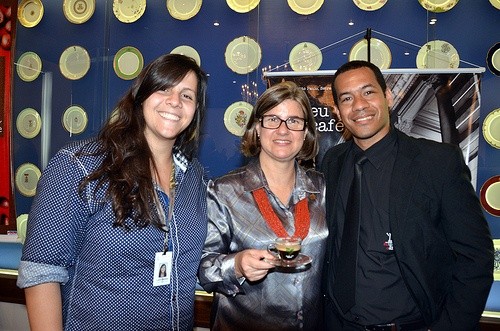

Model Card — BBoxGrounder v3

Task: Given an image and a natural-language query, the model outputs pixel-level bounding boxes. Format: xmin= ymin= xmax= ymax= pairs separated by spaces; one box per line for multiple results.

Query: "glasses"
xmin=258 ymin=115 xmax=310 ymax=131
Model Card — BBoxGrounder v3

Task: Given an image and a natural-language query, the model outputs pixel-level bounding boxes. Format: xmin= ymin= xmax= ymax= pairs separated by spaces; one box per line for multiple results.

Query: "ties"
xmin=333 ymin=147 xmax=369 ymax=313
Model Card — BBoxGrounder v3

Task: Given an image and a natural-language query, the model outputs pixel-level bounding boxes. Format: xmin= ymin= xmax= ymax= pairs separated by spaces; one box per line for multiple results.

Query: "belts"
xmin=323 ymin=290 xmax=425 ymax=331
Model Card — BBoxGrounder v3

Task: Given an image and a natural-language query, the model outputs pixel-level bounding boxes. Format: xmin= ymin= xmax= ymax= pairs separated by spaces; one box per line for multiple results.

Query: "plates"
xmin=486 ymin=42 xmax=500 ymax=77
xmin=264 ymin=253 xmax=311 ymax=268
xmin=16 ymin=52 xmax=42 ymax=82
xmin=167 ymin=0 xmax=202 ymax=21
xmin=59 ymin=46 xmax=90 ymax=80
xmin=17 ymin=214 xmax=31 ymax=245
xmin=170 ymin=45 xmax=200 ymax=68
xmin=354 ymin=1 xmax=388 ymax=11
xmin=491 ymin=238 xmax=500 ymax=281
xmin=227 ymin=0 xmax=261 ymax=13
xmin=288 ymin=42 xmax=323 ymax=72
xmin=16 ymin=108 xmax=42 ymax=139
xmin=60 ymin=104 xmax=88 ymax=136
xmin=416 ymin=41 xmax=459 ymax=69
xmin=224 ymin=101 xmax=254 ymax=136
xmin=482 ymin=108 xmax=500 ymax=149
xmin=14 ymin=163 xmax=41 ymax=197
xmin=17 ymin=0 xmax=44 ymax=28
xmin=480 ymin=176 xmax=500 ymax=218
xmin=419 ymin=0 xmax=459 ymax=13
xmin=113 ymin=0 xmax=146 ymax=23
xmin=63 ymin=0 xmax=95 ymax=25
xmin=112 ymin=46 xmax=144 ymax=80
xmin=224 ymin=36 xmax=262 ymax=74
xmin=348 ymin=37 xmax=391 ymax=70
xmin=287 ymin=0 xmax=324 ymax=15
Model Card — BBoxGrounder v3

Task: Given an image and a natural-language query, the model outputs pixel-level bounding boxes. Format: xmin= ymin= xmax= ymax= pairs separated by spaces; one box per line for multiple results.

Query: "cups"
xmin=267 ymin=236 xmax=301 ymax=263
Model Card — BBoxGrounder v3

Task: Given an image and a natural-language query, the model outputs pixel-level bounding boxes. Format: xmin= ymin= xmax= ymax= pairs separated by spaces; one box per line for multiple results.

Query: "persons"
xmin=199 ymin=81 xmax=329 ymax=331
xmin=17 ymin=53 xmax=206 ymax=330
xmin=318 ymin=60 xmax=495 ymax=331
